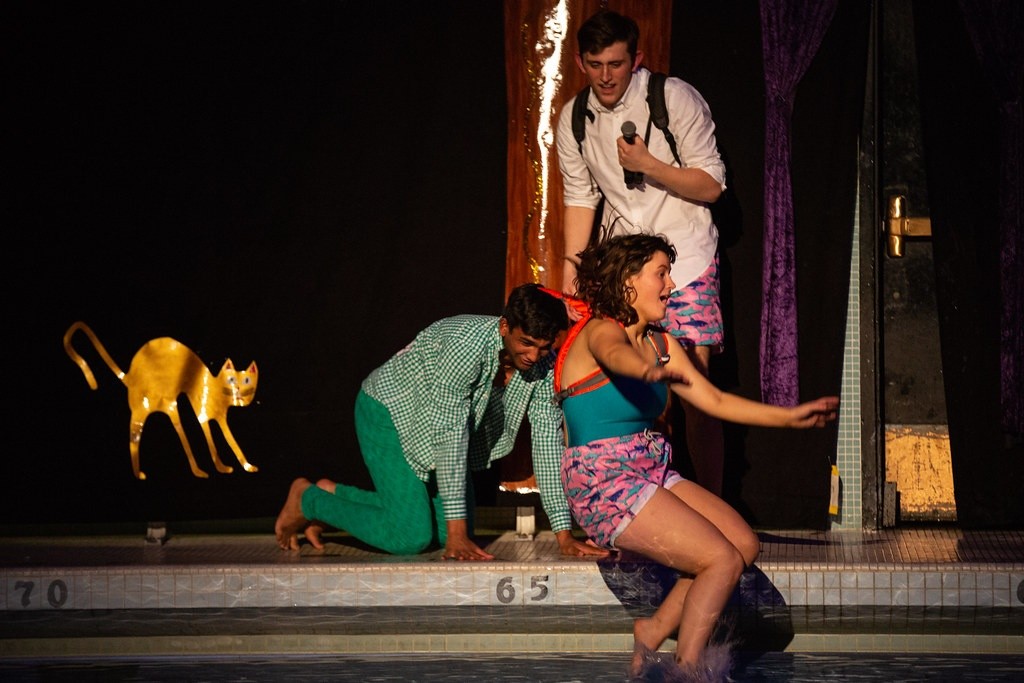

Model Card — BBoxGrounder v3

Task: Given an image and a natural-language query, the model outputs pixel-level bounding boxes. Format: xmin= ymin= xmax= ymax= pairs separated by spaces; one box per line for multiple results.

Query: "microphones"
xmin=622 ymin=120 xmax=637 ymax=185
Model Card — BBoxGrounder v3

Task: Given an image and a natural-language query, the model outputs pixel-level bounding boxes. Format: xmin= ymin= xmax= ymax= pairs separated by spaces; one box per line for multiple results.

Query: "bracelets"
xmin=642 ymin=364 xmax=654 ymax=382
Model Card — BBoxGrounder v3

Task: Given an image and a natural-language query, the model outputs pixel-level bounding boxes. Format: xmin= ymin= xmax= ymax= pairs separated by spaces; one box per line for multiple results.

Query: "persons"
xmin=553 ymin=7 xmax=727 ymax=502
xmin=560 ymin=230 xmax=839 ymax=682
xmin=274 ymin=279 xmax=612 ymax=563
